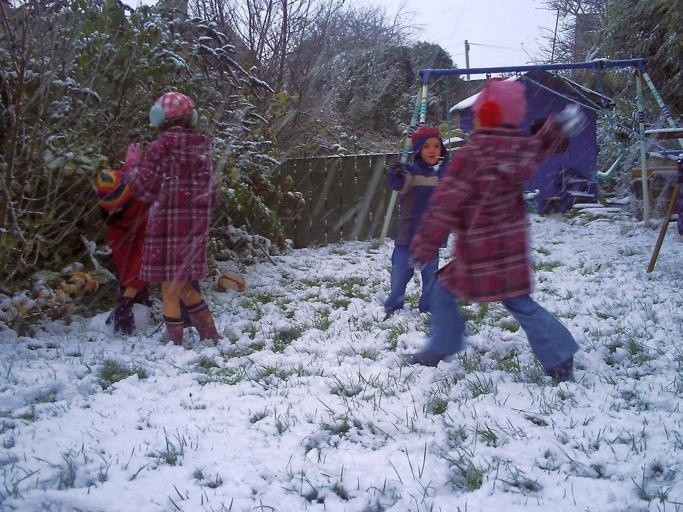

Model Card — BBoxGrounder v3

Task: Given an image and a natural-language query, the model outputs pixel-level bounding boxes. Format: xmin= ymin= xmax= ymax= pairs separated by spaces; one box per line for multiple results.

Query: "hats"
xmin=154 ymin=91 xmax=195 ymax=119
xmin=412 ymin=126 xmax=447 ymax=164
xmin=96 ymin=169 xmax=134 ymax=209
xmin=470 ymin=79 xmax=528 ymax=128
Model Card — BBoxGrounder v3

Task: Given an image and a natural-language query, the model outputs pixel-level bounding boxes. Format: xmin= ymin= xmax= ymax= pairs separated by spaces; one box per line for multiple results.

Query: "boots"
xmin=133 ymin=286 xmax=153 ymax=308
xmin=105 ymin=296 xmax=136 ymax=336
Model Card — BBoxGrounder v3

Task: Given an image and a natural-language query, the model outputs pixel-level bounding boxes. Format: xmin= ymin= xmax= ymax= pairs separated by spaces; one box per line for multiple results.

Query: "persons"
xmin=409 ymin=79 xmax=580 ymax=383
xmin=129 ymin=93 xmax=221 ymax=347
xmin=94 ymin=142 xmax=152 ymax=333
xmin=385 ymin=126 xmax=449 ymax=318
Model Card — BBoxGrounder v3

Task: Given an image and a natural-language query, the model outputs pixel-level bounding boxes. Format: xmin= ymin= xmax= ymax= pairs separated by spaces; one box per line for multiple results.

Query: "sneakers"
xmin=551 ymin=356 xmax=574 ymax=387
xmin=411 ymin=353 xmax=447 ymax=367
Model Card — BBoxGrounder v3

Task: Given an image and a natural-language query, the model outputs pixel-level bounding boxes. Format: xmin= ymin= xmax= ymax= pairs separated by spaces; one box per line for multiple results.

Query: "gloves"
xmin=124 ymin=143 xmax=142 ymax=167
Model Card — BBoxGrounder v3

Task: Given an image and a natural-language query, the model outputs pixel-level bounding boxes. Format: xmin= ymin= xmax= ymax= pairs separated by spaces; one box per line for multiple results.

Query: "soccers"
xmin=219 ymin=270 xmax=245 ymax=294
